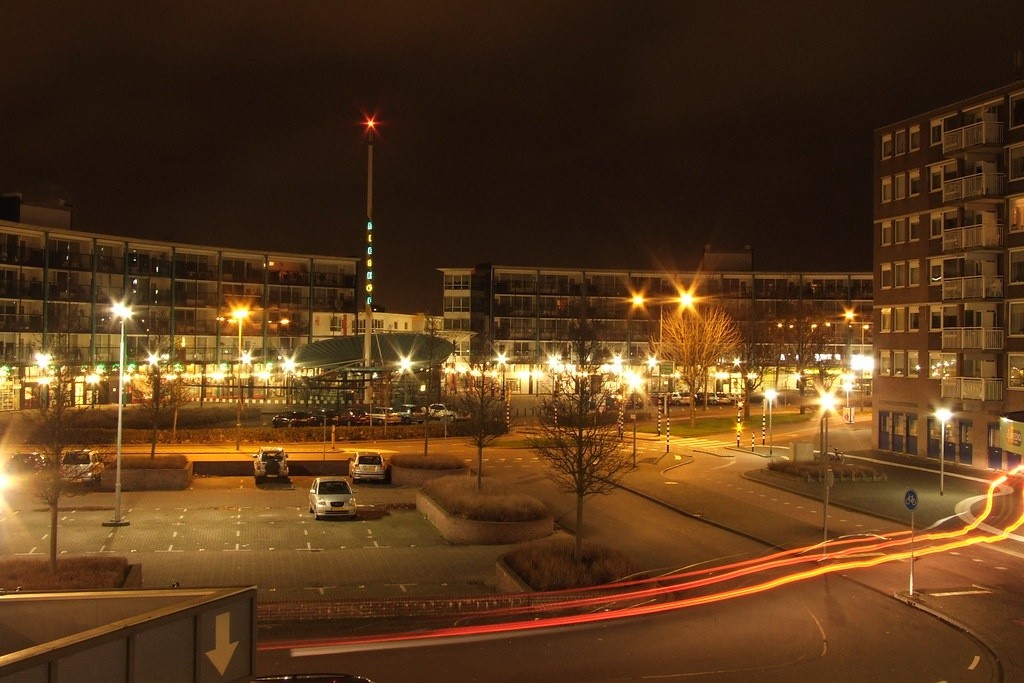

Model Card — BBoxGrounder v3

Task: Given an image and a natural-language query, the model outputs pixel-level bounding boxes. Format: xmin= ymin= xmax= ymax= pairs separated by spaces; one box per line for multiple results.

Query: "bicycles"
xmin=828 ymin=445 xmax=846 ymax=465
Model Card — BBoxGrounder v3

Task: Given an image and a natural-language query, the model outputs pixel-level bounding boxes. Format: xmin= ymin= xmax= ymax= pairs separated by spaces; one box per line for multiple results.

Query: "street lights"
xmin=842 ymin=381 xmax=852 ymax=408
xmin=231 ymin=304 xmax=247 ymax=454
xmin=629 ymin=374 xmax=639 ymax=467
xmin=764 ymin=390 xmax=778 ymax=455
xmin=817 ymin=395 xmax=834 ymax=452
xmin=101 ymin=302 xmax=137 ymax=527
xmin=497 ymin=354 xmax=508 ymax=399
xmin=935 ymin=409 xmax=948 ymax=497
xmin=532 ymin=371 xmax=540 ymax=398
xmin=647 ymin=357 xmax=658 ymax=405
xmin=400 ymin=360 xmax=413 ymax=414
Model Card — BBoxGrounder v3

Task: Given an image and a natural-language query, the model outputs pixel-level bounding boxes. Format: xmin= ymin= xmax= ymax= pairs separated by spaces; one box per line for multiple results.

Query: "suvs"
xmin=252 ymin=446 xmax=291 ymax=483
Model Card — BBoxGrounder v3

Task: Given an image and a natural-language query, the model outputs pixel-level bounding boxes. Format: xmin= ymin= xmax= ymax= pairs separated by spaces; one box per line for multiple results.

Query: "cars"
xmin=339 ymin=408 xmax=372 ymax=427
xmin=4 ymin=452 xmax=50 ymax=478
xmin=368 ymin=407 xmax=401 ymax=426
xmin=625 ymin=392 xmax=740 ymax=408
xmin=395 ymin=402 xmax=426 ymax=425
xmin=271 ymin=410 xmax=318 ymax=428
xmin=307 ymin=476 xmax=358 ymax=519
xmin=349 ymin=449 xmax=387 ymax=483
xmin=425 ymin=403 xmax=473 ymax=421
xmin=309 ymin=410 xmax=342 ymax=425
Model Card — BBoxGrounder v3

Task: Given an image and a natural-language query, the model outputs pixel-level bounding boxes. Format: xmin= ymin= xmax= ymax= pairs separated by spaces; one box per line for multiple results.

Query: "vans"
xmin=55 ymin=449 xmax=102 ymax=487
